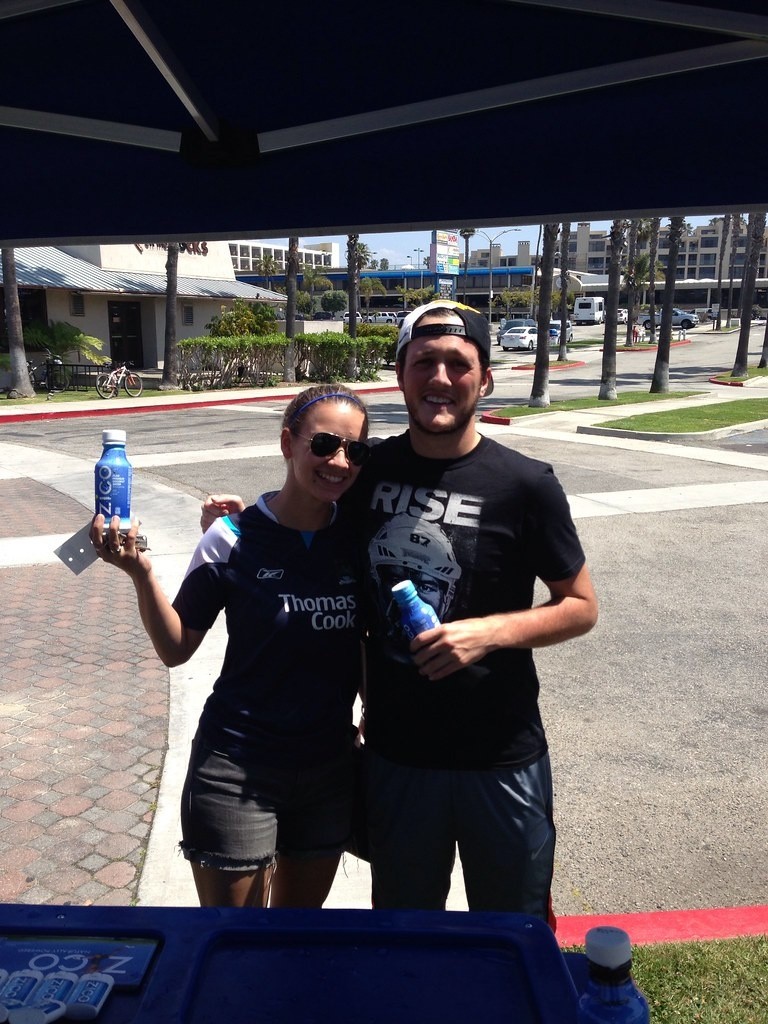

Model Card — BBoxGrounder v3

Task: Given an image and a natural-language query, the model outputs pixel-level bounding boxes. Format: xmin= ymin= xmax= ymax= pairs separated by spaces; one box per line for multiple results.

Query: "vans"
xmin=549 ymin=320 xmax=573 ymax=344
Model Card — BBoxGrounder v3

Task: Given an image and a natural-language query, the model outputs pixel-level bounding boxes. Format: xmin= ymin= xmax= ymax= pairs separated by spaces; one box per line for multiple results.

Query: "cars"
xmin=689 ymin=309 xmax=714 ymax=322
xmin=311 ymin=312 xmax=334 ymax=320
xmin=502 ymin=327 xmax=538 ymax=351
xmin=617 ymin=309 xmax=628 ymax=323
xmin=340 ymin=311 xmax=409 ymax=327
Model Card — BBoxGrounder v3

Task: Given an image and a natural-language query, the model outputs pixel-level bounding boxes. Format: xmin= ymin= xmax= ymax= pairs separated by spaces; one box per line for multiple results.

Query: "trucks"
xmin=574 ymin=297 xmax=605 ymax=324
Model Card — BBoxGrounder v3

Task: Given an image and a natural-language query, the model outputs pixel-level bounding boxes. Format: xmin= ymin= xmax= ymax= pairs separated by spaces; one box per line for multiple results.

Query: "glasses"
xmin=291 ymin=434 xmax=375 ymax=469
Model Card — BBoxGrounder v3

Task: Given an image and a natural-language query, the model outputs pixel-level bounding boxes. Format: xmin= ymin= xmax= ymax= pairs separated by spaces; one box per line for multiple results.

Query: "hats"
xmin=395 ymin=302 xmax=492 ymax=361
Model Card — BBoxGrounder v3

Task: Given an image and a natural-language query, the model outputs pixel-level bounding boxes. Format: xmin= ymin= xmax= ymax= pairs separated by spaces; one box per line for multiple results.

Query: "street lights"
xmin=415 ymin=247 xmax=424 ymax=269
xmin=408 ymin=256 xmax=412 ymax=266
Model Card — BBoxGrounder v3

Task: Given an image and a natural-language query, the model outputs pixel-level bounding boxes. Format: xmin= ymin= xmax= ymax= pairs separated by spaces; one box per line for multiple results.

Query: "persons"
xmin=89 ymin=300 xmax=599 ymax=925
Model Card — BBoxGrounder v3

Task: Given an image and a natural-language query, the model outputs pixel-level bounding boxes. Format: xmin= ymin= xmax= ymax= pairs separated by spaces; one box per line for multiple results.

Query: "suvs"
xmin=497 ymin=318 xmax=536 ymax=345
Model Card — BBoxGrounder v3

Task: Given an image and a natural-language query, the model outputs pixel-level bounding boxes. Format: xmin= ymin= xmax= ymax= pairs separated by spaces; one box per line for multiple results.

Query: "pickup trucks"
xmin=638 ymin=307 xmax=700 ymax=329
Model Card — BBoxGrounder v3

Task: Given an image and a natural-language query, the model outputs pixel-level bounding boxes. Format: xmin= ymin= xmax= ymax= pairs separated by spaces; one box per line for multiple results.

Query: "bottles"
xmin=95 ymin=430 xmax=132 ymax=533
xmin=391 ymin=580 xmax=441 ymax=641
xmin=574 ymin=927 xmax=650 ymax=1024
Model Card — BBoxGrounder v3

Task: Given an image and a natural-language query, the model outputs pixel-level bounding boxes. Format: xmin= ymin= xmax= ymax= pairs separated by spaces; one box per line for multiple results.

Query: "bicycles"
xmin=27 ymin=347 xmax=70 ymax=394
xmin=95 ymin=361 xmax=143 ymax=399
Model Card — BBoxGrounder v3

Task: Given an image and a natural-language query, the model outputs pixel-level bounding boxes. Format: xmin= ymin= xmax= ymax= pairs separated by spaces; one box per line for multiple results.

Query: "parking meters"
xmin=474 ymin=228 xmax=522 ymax=329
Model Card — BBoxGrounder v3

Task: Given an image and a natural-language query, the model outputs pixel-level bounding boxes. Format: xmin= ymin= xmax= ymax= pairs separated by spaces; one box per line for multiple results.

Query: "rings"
xmin=111 ymin=546 xmax=120 ymax=553
